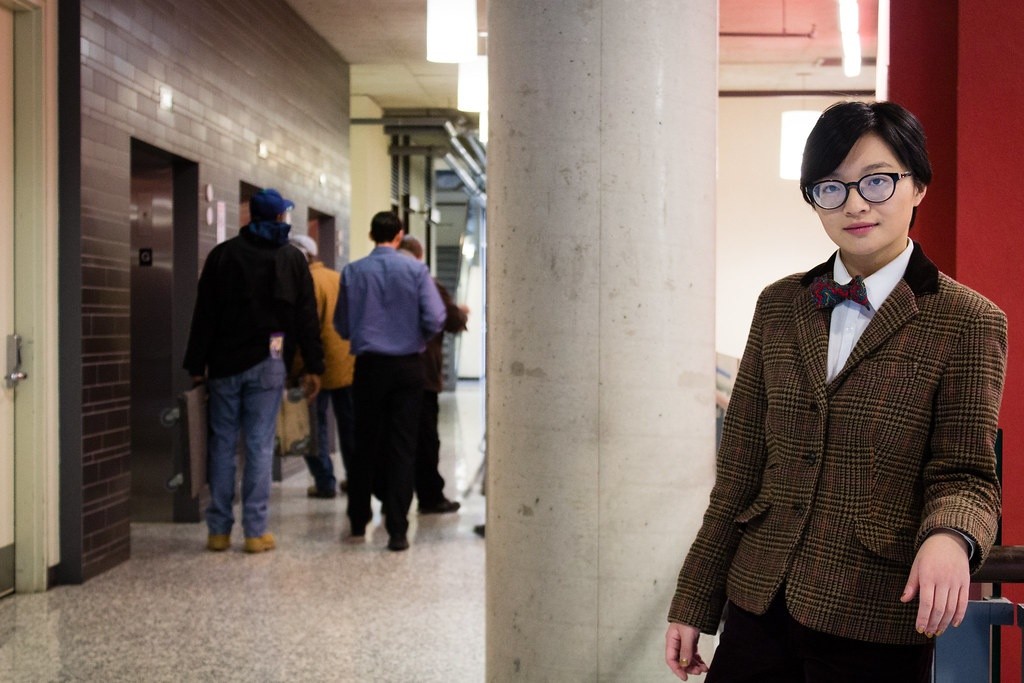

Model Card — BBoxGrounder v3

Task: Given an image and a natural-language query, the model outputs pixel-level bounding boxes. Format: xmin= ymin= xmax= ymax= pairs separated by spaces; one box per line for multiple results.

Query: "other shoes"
xmin=307 ymin=484 xmax=337 ymax=498
xmin=342 ymin=481 xmax=346 ymax=492
xmin=209 ymin=532 xmax=230 ymax=550
xmin=351 ymin=520 xmax=366 ymax=537
xmin=389 ymin=534 xmax=408 ymax=551
xmin=243 ymin=535 xmax=273 ymax=551
xmin=419 ymin=497 xmax=459 ymax=513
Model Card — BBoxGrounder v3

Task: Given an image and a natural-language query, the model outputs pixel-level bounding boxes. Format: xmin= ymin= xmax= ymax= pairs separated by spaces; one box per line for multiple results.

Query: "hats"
xmin=249 ymin=190 xmax=295 ymax=222
xmin=288 ymin=234 xmax=318 ymax=256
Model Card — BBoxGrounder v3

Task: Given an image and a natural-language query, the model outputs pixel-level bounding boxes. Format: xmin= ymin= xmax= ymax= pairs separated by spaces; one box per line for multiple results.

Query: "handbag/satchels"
xmin=279 ymin=388 xmax=313 ymax=455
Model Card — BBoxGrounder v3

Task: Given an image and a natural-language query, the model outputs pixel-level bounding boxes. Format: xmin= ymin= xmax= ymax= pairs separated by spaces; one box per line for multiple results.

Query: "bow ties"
xmin=812 ymin=274 xmax=871 ymax=311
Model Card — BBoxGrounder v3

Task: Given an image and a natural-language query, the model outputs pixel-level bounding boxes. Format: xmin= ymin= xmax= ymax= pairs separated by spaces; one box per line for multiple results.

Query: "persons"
xmin=396 ymin=236 xmax=470 ymax=516
xmin=180 ymin=187 xmax=322 ymax=552
xmin=287 ymin=231 xmax=354 ymax=496
xmin=472 ymin=524 xmax=486 ymax=538
xmin=330 ymin=211 xmax=447 ymax=551
xmin=664 ymin=103 xmax=1008 ymax=683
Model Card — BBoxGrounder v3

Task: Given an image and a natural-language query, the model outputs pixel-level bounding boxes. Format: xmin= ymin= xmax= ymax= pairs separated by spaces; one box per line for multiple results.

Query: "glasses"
xmin=808 ymin=171 xmax=913 ymax=210
